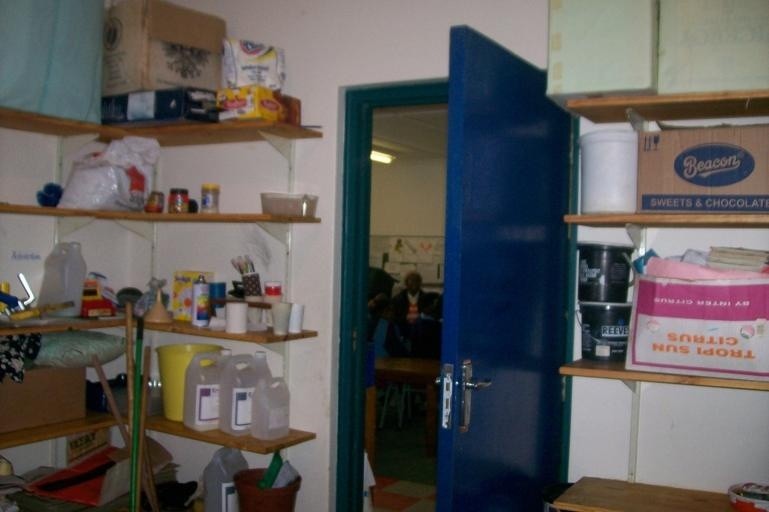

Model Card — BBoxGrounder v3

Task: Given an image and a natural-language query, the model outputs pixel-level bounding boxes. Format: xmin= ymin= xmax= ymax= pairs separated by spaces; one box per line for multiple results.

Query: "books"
xmin=632 ymin=244 xmax=768 ymax=280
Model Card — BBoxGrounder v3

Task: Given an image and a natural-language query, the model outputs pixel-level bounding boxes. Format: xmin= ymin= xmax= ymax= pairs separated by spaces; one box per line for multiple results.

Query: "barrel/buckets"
xmin=38 ymin=241 xmax=87 ymax=317
xmin=155 ymin=342 xmax=223 ymax=422
xmin=219 ymin=351 xmax=273 ymax=436
xmin=576 ymin=242 xmax=635 ymax=303
xmin=233 ymin=468 xmax=303 ymax=512
xmin=251 ymin=377 xmax=290 ymax=440
xmin=184 ymin=348 xmax=233 ymax=431
xmin=578 ymin=301 xmax=632 ymax=363
xmin=579 ymin=130 xmax=637 ymax=215
xmin=204 ymin=442 xmax=249 ymax=512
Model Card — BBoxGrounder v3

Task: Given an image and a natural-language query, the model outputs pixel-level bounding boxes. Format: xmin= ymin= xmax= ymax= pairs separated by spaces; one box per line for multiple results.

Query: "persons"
xmin=409 ymin=292 xmax=442 ymax=358
xmin=388 ymin=271 xmax=427 ymax=357
xmin=369 ymin=267 xmax=392 ymax=382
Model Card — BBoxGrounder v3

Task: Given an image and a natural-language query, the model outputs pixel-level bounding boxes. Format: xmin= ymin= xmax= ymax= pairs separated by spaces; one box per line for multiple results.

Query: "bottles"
xmin=145 ymin=191 xmax=165 ymax=214
xmin=167 ymin=187 xmax=189 ymax=213
xmin=264 ymin=281 xmax=282 ymax=296
xmin=200 ymin=182 xmax=220 ymax=213
xmin=188 ymin=198 xmax=199 ymax=213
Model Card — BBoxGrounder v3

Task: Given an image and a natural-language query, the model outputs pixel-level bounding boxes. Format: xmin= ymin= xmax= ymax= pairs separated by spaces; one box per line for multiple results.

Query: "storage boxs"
xmin=101 ymin=1 xmax=226 ymax=98
xmin=636 ymin=125 xmax=768 ymax=214
xmin=100 ymin=85 xmax=218 ymax=128
xmin=0 ymin=368 xmax=86 ymax=434
xmin=216 ymin=85 xmax=288 ymax=122
xmin=624 ymin=270 xmax=768 ymax=381
